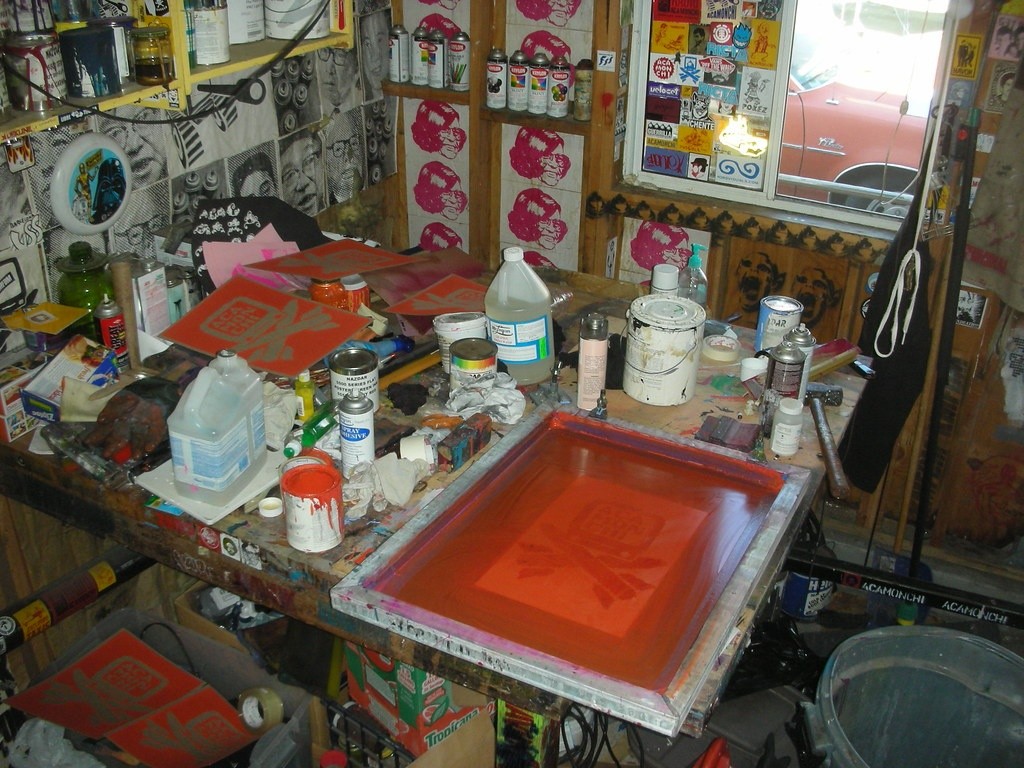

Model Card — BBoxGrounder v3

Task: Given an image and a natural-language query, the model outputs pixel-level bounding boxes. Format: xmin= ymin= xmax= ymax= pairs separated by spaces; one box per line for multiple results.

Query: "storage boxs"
xmin=344 ymin=638 xmax=493 ymax=756
xmin=19 ymin=334 xmax=121 ymax=423
xmin=0 ymin=407 xmax=43 ymax=444
xmin=0 ymin=337 xmax=69 ymax=417
xmin=22 ymin=605 xmax=313 ymax=768
xmin=172 ymin=580 xmax=253 ymax=656
xmin=320 ymin=675 xmax=416 ymax=768
xmin=625 ymin=686 xmax=813 ymax=768
xmin=496 ymin=698 xmax=560 ymax=768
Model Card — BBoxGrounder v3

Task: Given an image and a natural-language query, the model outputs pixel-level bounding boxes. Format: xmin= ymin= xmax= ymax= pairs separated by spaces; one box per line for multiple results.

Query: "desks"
xmin=0 ymin=269 xmax=875 ymax=739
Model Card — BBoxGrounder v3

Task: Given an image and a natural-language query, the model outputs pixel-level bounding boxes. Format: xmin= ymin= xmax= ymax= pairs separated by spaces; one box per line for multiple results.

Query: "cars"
xmin=776 ymin=61 xmax=929 ymax=218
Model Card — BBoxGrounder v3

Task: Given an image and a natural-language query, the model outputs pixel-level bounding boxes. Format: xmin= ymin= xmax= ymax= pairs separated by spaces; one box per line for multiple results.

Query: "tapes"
xmin=238 ymin=688 xmax=284 ymax=734
xmin=703 ymin=331 xmax=740 ymax=361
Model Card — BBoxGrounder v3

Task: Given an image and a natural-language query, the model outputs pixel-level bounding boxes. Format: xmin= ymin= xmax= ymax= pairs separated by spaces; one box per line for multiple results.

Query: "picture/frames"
xmin=330 ymin=399 xmax=812 ymax=737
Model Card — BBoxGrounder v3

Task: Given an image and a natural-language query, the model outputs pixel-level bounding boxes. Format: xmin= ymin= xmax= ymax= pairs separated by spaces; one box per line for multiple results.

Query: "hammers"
xmin=801 ymin=380 xmax=854 ymax=492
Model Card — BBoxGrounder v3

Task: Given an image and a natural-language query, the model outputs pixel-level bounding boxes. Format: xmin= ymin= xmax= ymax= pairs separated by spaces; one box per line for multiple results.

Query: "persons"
xmin=28 ymin=125 xmax=84 ymax=230
xmin=115 ymin=187 xmax=171 ymax=269
xmin=315 ymin=10 xmax=392 ymax=120
xmin=232 ymin=113 xmax=366 ymax=218
xmin=95 ymin=104 xmax=169 ymax=193
xmin=242 ymin=542 xmax=262 ymax=570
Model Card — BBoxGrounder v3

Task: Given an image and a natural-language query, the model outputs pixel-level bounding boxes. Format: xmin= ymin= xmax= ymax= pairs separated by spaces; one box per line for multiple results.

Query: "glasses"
xmin=281 ymin=152 xmax=321 ymax=188
xmin=115 ymin=214 xmax=164 ymax=244
xmin=326 ymin=135 xmax=361 ymax=156
xmin=105 ymin=107 xmax=156 ymax=150
xmin=319 ymin=46 xmax=348 ymax=66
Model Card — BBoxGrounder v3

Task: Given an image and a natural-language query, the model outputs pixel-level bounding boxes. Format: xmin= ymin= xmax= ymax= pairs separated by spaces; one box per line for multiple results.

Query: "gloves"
xmin=78 ymin=377 xmax=185 ymax=472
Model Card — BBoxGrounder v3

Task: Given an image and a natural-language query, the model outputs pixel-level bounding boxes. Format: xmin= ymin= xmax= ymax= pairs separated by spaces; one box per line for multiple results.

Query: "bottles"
xmin=577 ymin=313 xmax=609 ymax=412
xmin=339 ymin=388 xmax=375 ymax=479
xmin=94 ymin=293 xmax=130 ymax=373
xmin=216 ymin=601 xmax=244 ymax=633
xmin=760 ymin=321 xmax=816 ymax=457
xmin=309 ymin=277 xmax=371 ymax=316
xmin=132 ymin=25 xmax=174 ymax=88
xmin=54 ymin=241 xmax=114 ymax=343
xmin=284 ymin=400 xmax=337 ymax=458
xmin=388 ymin=24 xmax=594 ymax=121
xmin=650 ymin=264 xmax=679 ymax=298
xmin=292 ymin=369 xmax=330 ymax=423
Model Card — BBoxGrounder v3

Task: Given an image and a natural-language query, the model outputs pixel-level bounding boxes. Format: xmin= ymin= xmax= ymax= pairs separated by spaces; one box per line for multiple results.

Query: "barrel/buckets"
xmin=754 ymin=295 xmax=804 ymax=354
xmin=483 ymin=248 xmax=555 ymax=386
xmin=166 ymin=348 xmax=268 ymax=507
xmin=783 ymin=541 xmax=840 ymax=621
xmin=0 ymin=0 xmax=331 ymax=125
xmin=619 ymin=295 xmax=706 ymax=408
xmin=800 ymin=626 xmax=1024 ymax=768
xmin=329 ymin=346 xmax=380 ymax=415
xmin=433 ymin=313 xmax=498 ymax=396
xmin=278 ymin=456 xmax=345 ymax=553
